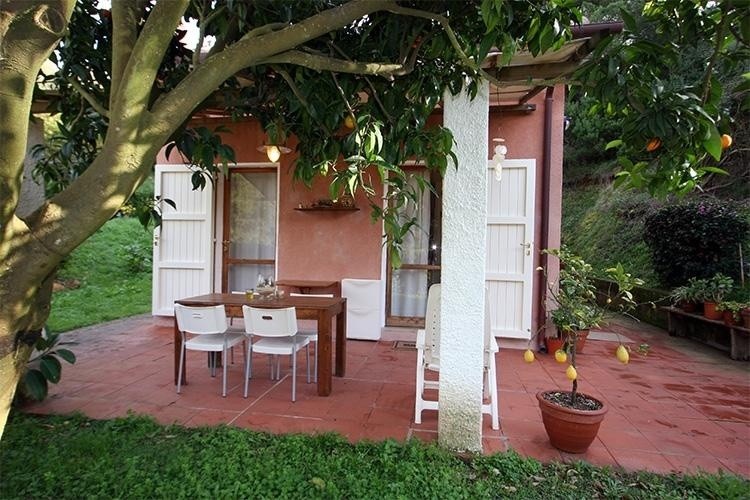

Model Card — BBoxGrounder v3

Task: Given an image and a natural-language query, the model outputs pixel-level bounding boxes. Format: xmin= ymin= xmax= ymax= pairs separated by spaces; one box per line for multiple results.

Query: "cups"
xmin=245 ymin=273 xmax=286 ymax=301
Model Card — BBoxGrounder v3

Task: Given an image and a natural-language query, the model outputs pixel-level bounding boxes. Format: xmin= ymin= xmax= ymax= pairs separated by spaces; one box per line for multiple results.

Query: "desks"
xmin=171 ymin=291 xmax=347 ymax=396
xmin=272 ymin=279 xmax=338 ymax=295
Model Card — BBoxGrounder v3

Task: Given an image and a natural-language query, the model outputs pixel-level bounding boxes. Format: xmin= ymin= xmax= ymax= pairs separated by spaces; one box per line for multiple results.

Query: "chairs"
xmin=173 ymin=301 xmax=249 ymax=400
xmin=241 ymin=303 xmax=313 ymax=404
xmin=271 ymin=293 xmax=334 ymax=384
xmin=213 ymin=291 xmax=262 ymax=377
xmin=415 ymin=281 xmax=502 ymax=432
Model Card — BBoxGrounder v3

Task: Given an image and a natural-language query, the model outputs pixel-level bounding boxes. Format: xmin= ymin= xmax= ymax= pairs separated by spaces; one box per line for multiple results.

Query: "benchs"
xmin=660 ymin=304 xmax=750 ymax=363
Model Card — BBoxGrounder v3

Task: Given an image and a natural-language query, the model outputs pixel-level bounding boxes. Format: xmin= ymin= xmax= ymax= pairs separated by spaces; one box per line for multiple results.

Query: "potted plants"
xmin=667 ymin=274 xmax=749 ymax=330
xmin=520 ymin=241 xmax=656 ymax=455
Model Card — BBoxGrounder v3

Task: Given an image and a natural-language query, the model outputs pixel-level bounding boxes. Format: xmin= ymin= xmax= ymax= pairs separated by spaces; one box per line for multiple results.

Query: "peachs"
xmin=346 ymin=115 xmax=356 ymax=128
xmin=722 ymin=135 xmax=732 ymax=148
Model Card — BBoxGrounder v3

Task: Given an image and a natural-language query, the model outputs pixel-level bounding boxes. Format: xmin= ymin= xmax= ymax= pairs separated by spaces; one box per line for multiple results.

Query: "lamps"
xmin=257 ymin=137 xmax=293 ymax=165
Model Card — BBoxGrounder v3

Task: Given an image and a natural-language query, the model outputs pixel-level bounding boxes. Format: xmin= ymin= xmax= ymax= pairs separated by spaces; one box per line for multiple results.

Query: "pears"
xmin=565 ymin=364 xmax=577 ymax=380
xmin=617 ymin=346 xmax=629 ymax=364
xmin=556 ymin=349 xmax=566 ymax=363
xmin=525 ymin=351 xmax=534 ymax=362
